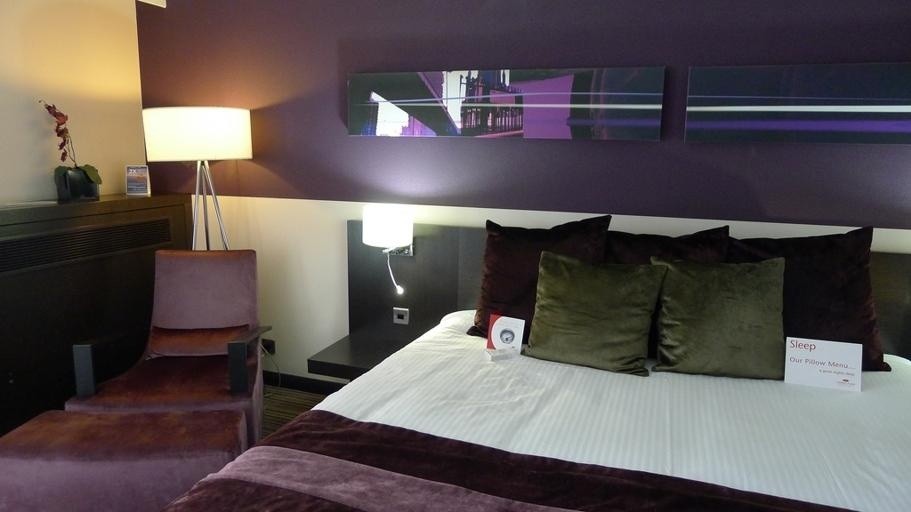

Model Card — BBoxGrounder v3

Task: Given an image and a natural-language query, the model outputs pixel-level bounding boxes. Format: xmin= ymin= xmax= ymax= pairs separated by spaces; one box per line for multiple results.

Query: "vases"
xmin=56 ymin=167 xmax=101 ymax=203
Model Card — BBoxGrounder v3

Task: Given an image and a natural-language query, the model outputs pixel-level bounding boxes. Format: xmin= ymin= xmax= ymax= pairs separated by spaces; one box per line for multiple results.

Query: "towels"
xmin=162 ymin=408 xmax=863 ymax=512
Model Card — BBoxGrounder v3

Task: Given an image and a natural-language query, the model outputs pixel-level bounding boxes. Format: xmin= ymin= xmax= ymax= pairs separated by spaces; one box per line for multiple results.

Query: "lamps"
xmin=141 ymin=105 xmax=255 ymax=251
xmin=360 ymin=202 xmax=415 ymax=297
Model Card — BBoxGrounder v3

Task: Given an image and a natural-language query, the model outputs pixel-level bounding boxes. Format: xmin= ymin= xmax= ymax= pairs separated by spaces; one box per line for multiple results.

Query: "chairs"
xmin=65 ymin=249 xmax=276 ymax=450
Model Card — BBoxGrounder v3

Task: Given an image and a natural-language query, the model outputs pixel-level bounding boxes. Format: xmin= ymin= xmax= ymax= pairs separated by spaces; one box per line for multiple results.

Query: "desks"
xmin=0 ymin=193 xmax=193 ymax=436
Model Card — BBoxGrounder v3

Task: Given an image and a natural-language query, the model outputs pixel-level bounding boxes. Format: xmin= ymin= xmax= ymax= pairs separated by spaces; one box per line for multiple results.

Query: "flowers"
xmin=34 ymin=93 xmax=103 ymax=186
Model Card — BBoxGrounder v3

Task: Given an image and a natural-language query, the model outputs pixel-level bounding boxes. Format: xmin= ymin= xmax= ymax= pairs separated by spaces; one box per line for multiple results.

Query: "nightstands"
xmin=305 ymin=219 xmax=459 ymax=388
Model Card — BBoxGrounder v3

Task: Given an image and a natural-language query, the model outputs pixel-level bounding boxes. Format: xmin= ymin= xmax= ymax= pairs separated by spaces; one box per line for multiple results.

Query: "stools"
xmin=0 ymin=410 xmax=249 ymax=512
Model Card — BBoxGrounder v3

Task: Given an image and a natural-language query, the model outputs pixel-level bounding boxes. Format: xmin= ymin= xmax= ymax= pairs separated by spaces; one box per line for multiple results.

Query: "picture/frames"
xmin=124 ymin=164 xmax=151 ymax=196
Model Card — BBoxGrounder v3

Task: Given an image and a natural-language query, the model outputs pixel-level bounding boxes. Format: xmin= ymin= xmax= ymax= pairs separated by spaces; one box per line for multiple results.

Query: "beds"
xmin=163 ymin=220 xmax=911 ymax=512
